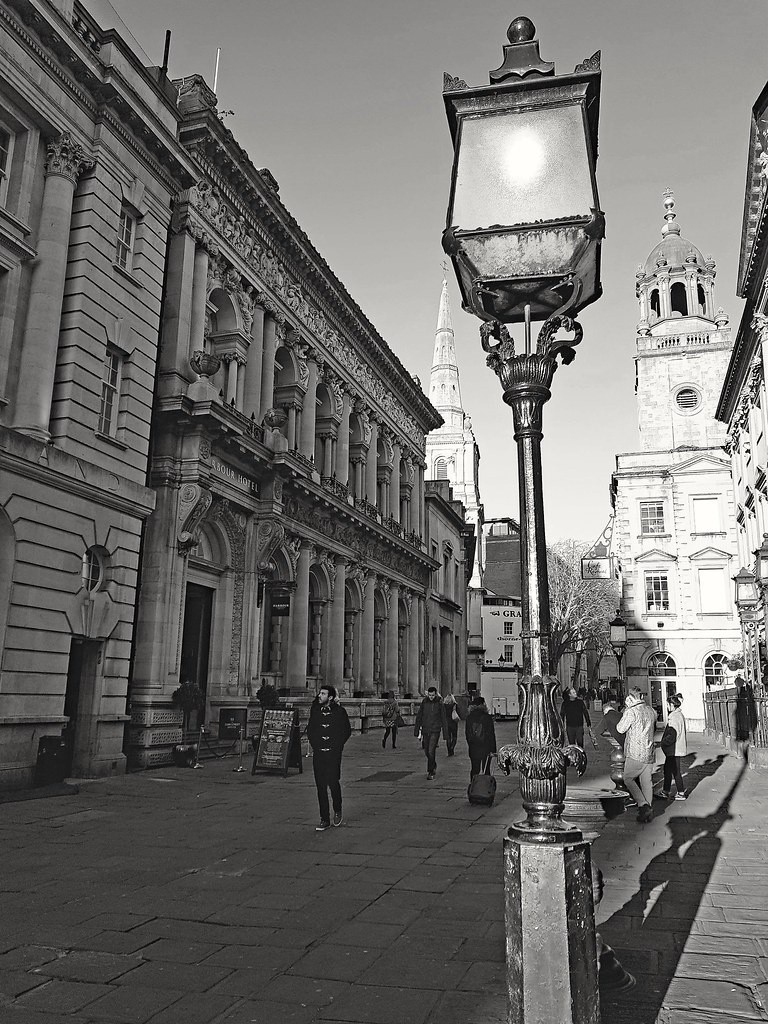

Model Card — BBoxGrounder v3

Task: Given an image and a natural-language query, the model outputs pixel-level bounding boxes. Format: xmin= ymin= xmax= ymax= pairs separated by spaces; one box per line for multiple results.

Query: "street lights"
xmin=438 ymin=13 xmax=613 ymax=1024
xmin=731 ymin=568 xmax=759 ymax=690
xmin=756 ymin=532 xmax=768 ymax=654
xmin=609 ymin=609 xmax=627 ymax=712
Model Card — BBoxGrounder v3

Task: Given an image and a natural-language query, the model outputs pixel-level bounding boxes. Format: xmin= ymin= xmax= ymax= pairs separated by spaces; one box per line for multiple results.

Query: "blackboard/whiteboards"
xmin=253 ymin=708 xmax=298 ymax=770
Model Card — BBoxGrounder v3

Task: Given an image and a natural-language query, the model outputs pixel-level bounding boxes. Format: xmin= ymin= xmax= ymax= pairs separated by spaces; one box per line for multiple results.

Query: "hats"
xmin=473 ymin=697 xmax=485 ymax=705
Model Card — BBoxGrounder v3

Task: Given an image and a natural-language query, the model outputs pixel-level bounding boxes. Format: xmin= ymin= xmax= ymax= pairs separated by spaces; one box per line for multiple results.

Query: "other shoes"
xmin=333 ymin=811 xmax=342 ymax=826
xmin=654 ymin=791 xmax=669 ymax=799
xmin=670 ymin=793 xmax=686 ymax=800
xmin=433 ymin=769 xmax=436 ymax=775
xmin=392 ymin=742 xmax=397 ymax=749
xmin=637 ymin=806 xmax=651 ymax=821
xmin=316 ymin=820 xmax=330 ymax=830
xmin=427 ymin=774 xmax=433 ymax=779
xmin=382 ymin=739 xmax=385 ymax=749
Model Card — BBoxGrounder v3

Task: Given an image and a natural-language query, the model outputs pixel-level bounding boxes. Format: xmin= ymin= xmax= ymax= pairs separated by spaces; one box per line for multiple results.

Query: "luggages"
xmin=468 ymin=754 xmax=497 ymax=805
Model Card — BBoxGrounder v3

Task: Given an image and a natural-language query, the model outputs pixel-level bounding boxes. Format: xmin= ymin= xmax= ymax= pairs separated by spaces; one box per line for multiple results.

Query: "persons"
xmin=616 ymin=686 xmax=659 ymax=824
xmin=464 ymin=697 xmax=497 ymax=783
xmin=561 ymin=686 xmax=635 ymax=712
xmin=593 ymin=701 xmax=641 ymax=807
xmin=442 ymin=693 xmax=459 ymax=756
xmin=560 ymin=687 xmax=591 ymax=750
xmin=653 ymin=693 xmax=688 ymax=801
xmin=381 ymin=691 xmax=400 ymax=750
xmin=307 ymin=686 xmax=352 ymax=830
xmin=414 ymin=687 xmax=448 ymax=781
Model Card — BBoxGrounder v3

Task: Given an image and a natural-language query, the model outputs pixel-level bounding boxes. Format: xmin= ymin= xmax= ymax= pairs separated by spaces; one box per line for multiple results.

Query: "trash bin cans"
xmin=36 ymin=735 xmax=69 ymax=784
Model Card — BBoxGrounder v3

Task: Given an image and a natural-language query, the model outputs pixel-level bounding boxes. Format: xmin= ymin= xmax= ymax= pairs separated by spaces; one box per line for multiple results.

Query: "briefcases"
xmin=588 ymin=726 xmax=601 ymax=749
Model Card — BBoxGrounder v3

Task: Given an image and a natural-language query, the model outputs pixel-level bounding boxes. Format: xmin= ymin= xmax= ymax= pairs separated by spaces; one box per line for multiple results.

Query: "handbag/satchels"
xmin=452 ymin=705 xmax=460 ymax=722
xmin=396 ymin=710 xmax=404 ymax=727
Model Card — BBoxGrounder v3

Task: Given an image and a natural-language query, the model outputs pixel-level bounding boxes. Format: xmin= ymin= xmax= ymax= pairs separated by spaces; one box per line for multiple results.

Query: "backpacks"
xmin=468 ymin=715 xmax=488 ymax=745
xmin=382 ymin=704 xmax=394 ymax=718
